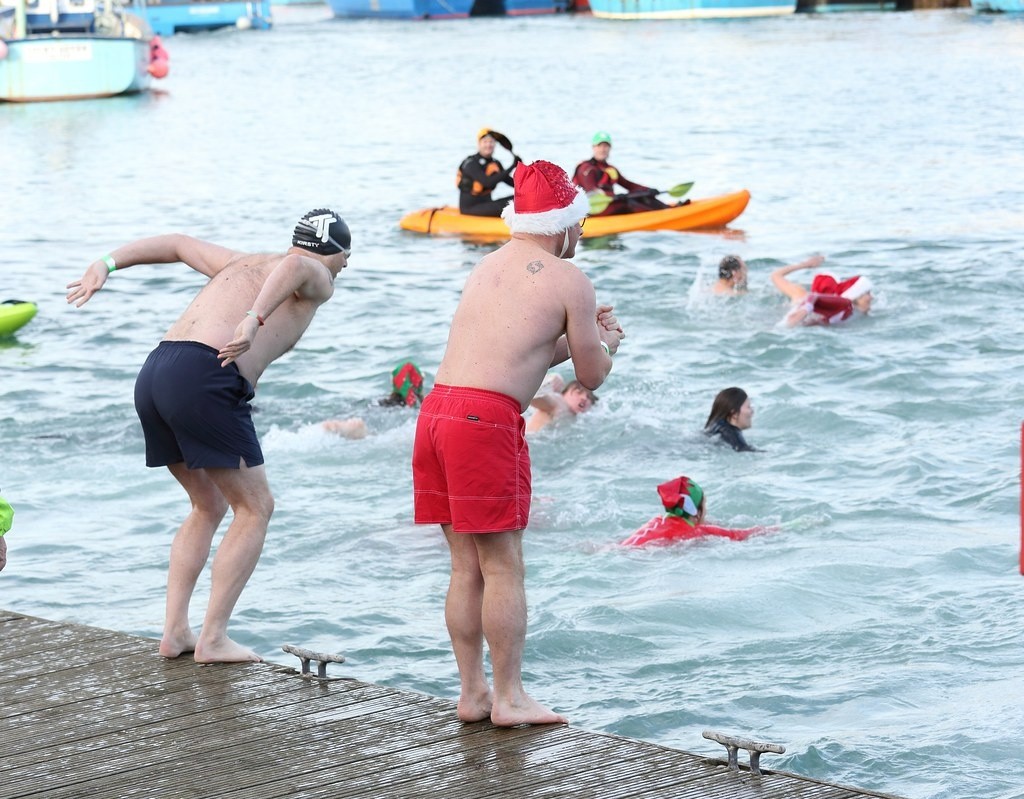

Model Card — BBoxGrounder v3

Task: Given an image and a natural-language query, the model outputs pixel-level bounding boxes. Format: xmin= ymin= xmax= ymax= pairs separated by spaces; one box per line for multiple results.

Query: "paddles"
xmin=487 ymin=130 xmax=517 ymax=158
xmin=585 ymin=181 xmax=695 ymax=215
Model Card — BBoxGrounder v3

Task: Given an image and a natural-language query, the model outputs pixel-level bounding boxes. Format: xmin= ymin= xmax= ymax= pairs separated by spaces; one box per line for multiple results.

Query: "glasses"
xmin=576 ymin=217 xmax=586 ymax=228
xmin=329 ymin=236 xmax=351 ymax=259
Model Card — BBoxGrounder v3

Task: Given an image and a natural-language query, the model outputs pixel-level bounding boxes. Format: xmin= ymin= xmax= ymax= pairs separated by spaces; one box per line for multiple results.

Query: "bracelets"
xmin=246 ymin=310 xmax=264 ymax=326
xmin=101 ymin=254 xmax=116 ymax=272
xmin=600 ymin=341 xmax=609 ymax=356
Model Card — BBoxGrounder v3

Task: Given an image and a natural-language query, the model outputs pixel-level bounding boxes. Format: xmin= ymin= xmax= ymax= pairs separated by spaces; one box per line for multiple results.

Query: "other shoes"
xmin=674 ymin=199 xmax=689 ymax=207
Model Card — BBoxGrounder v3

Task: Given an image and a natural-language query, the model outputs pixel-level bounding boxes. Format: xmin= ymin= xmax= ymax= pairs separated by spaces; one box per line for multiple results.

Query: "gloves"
xmin=514 ymin=156 xmax=522 ymax=165
xmin=613 ymin=194 xmax=626 ymax=205
xmin=648 ymin=188 xmax=660 ymax=197
xmin=486 ymin=178 xmax=496 ymax=190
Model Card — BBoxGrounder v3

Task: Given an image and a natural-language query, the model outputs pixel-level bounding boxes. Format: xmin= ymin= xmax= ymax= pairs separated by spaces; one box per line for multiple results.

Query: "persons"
xmin=65 ymin=208 xmax=351 ymax=663
xmin=523 ymin=373 xmax=600 ymax=434
xmin=456 ymin=129 xmax=522 ymax=217
xmin=572 ymin=132 xmax=691 ymax=218
xmin=411 ymin=159 xmax=624 ymax=726
xmin=623 ymin=475 xmax=779 ymax=549
xmin=703 ymin=387 xmax=758 ymax=451
xmin=717 ymin=253 xmax=873 ymax=331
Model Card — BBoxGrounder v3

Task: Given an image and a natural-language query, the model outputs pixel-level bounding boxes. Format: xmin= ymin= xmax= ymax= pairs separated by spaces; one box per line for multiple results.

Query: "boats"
xmin=400 ymin=189 xmax=750 ymax=242
xmin=0 ymin=0 xmax=152 ymax=102
xmin=970 ymin=0 xmax=1024 ymax=12
xmin=119 ymin=0 xmax=797 ymax=37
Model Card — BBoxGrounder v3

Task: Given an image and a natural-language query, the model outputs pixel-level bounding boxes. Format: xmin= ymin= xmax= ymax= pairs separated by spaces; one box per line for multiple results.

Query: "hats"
xmin=658 ymin=477 xmax=702 ymax=518
xmin=392 ymin=361 xmax=424 ymax=407
xmin=812 ymin=275 xmax=870 ymax=325
xmin=292 ymin=210 xmax=351 ymax=255
xmin=477 ymin=127 xmax=492 ymax=140
xmin=592 ymin=130 xmax=612 ymax=147
xmin=505 ymin=160 xmax=590 ymax=236
